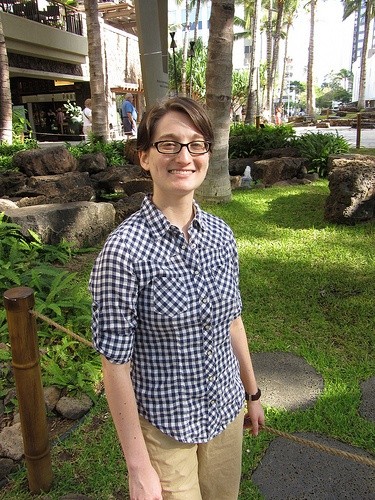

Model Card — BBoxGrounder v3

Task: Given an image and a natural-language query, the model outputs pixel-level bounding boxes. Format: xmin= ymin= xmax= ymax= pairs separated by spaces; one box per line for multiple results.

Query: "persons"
xmin=26 ymin=93 xmax=283 ymax=140
xmin=88 ymin=98 xmax=264 ymax=500
xmin=14 ymin=0 xmax=64 ymax=26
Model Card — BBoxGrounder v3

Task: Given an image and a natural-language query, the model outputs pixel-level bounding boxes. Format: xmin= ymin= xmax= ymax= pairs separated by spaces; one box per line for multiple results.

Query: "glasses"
xmin=148 ymin=139 xmax=212 ymax=156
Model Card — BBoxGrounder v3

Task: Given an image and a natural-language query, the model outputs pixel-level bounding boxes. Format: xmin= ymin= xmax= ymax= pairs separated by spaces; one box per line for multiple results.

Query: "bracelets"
xmin=245 ymin=388 xmax=261 ymax=401
